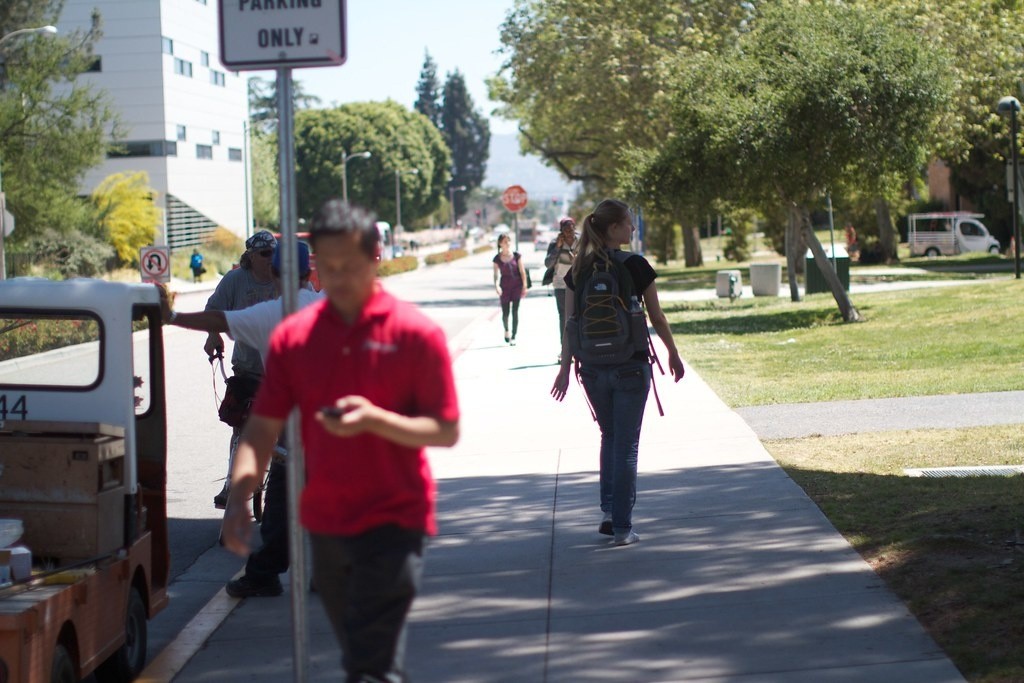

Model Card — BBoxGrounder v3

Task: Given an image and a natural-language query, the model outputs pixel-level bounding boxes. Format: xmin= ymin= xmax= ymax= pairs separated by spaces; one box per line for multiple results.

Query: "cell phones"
xmin=322 ymin=407 xmax=345 ymax=418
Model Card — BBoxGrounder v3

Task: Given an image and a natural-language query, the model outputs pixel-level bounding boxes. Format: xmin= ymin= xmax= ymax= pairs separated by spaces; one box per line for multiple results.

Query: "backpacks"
xmin=565 ymin=251 xmax=665 ymax=366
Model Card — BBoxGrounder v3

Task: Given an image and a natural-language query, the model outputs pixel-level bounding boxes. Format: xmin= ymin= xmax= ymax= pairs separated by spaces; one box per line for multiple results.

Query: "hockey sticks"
xmin=0 ymin=546 xmax=130 ymax=590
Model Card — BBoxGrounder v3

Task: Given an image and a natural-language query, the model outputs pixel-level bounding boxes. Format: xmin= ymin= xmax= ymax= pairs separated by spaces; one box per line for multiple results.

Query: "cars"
xmin=272 ymin=230 xmax=320 ymax=294
xmin=533 ymin=230 xmax=559 ymax=252
xmin=377 ymin=221 xmax=393 ymax=263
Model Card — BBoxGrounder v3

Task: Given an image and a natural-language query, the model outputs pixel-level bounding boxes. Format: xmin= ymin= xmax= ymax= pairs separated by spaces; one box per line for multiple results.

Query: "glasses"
xmin=258 ymin=250 xmax=274 ymax=257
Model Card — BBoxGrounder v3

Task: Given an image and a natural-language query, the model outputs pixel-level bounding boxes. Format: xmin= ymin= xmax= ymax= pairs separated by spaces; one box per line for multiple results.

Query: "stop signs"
xmin=500 ymin=184 xmax=527 ymax=213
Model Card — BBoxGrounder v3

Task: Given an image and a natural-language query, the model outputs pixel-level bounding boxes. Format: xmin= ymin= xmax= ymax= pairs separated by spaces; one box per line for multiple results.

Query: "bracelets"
xmin=169 ymin=312 xmax=176 ymax=322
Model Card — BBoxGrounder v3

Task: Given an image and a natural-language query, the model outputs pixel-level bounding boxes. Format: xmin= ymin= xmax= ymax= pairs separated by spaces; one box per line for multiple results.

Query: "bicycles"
xmin=207 ymin=350 xmax=274 ymax=522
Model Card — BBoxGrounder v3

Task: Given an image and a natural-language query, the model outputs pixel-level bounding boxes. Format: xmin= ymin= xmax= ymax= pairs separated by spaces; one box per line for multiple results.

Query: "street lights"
xmin=396 ymin=166 xmax=419 ymax=225
xmin=340 ymin=151 xmax=373 ymax=202
xmin=243 ymin=117 xmax=279 ymax=241
xmin=996 ymin=95 xmax=1022 ymax=281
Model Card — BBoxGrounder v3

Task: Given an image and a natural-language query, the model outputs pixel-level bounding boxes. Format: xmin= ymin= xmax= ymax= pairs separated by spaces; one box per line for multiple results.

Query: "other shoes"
xmin=598 ymin=511 xmax=615 ymax=535
xmin=226 ymin=566 xmax=282 ymax=597
xmin=510 ymin=342 xmax=517 ymax=346
xmin=615 ymin=530 xmax=640 ymax=544
xmin=214 ymin=483 xmax=230 ymax=506
xmin=505 ymin=333 xmax=511 ymax=343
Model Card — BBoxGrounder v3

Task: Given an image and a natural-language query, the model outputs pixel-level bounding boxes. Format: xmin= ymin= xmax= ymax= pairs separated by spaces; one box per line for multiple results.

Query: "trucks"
xmin=907 ymin=212 xmax=1000 ymax=258
xmin=0 ymin=277 xmax=172 ymax=683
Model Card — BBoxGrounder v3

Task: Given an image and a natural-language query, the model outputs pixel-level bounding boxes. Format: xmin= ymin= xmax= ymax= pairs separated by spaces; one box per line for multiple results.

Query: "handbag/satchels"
xmin=197 ymin=266 xmax=207 ymax=274
xmin=519 ymin=268 xmax=532 ymax=290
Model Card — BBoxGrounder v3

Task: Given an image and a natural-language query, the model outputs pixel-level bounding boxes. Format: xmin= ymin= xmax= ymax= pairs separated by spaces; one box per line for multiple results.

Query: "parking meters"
xmin=450 ymin=185 xmax=466 ymax=229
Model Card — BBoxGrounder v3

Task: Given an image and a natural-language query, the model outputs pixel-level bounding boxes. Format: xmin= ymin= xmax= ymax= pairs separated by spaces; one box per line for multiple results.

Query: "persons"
xmin=493 ymin=232 xmax=527 ymax=347
xmin=202 ymin=231 xmax=278 ymax=502
xmin=542 ymin=216 xmax=580 ymax=364
xmin=190 ymin=249 xmax=202 ymax=282
xmin=219 ymin=200 xmax=460 ymax=683
xmin=845 ymin=221 xmax=856 ymax=256
xmin=155 ymin=233 xmax=320 ymax=602
xmin=550 ymin=198 xmax=684 ymax=546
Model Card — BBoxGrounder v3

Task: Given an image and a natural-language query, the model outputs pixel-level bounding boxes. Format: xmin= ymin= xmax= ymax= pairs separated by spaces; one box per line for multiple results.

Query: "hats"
xmin=272 ymin=238 xmax=311 ymax=275
xmin=245 ymin=231 xmax=279 ymax=251
